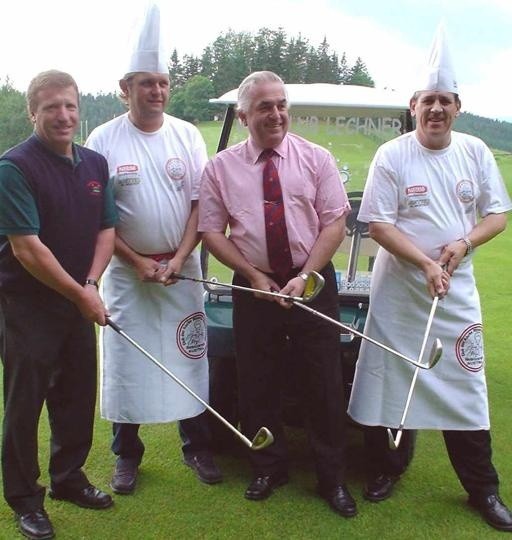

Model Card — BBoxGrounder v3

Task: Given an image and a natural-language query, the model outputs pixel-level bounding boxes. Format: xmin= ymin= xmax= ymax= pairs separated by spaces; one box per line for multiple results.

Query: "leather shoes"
xmin=49 ymin=484 xmax=114 ymax=509
xmin=15 ymin=509 xmax=54 ymax=540
xmin=319 ymin=483 xmax=357 ymax=516
xmin=245 ymin=472 xmax=288 ymax=500
xmin=468 ymin=493 xmax=512 ymax=531
xmin=363 ymin=472 xmax=400 ymax=500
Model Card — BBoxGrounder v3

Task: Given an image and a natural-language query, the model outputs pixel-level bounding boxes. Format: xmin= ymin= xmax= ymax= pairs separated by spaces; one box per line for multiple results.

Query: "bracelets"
xmin=456 ymin=237 xmax=472 ymax=257
xmin=298 ymin=273 xmax=309 ymax=281
xmin=82 ymin=279 xmax=99 ymax=289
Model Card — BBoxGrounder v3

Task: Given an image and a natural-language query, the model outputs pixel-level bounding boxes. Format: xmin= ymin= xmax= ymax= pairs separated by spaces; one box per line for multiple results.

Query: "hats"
xmin=414 ymin=40 xmax=459 ymax=94
xmin=127 ymin=4 xmax=169 ymax=74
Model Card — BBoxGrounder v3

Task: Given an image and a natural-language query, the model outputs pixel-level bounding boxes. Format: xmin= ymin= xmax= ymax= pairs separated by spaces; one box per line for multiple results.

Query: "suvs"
xmin=178 ymin=80 xmax=420 ymax=476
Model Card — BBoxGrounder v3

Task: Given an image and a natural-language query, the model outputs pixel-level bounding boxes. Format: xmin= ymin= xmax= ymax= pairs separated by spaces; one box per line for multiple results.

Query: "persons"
xmin=345 ymin=16 xmax=512 ymax=532
xmin=196 ymin=68 xmax=358 ymax=517
xmin=80 ymin=1 xmax=222 ymax=496
xmin=0 ymin=67 xmax=119 ymax=540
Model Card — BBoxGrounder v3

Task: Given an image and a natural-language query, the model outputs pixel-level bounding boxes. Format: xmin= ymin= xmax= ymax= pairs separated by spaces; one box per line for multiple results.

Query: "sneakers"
xmin=184 ymin=454 xmax=222 ymax=484
xmin=110 ymin=466 xmax=138 ymax=494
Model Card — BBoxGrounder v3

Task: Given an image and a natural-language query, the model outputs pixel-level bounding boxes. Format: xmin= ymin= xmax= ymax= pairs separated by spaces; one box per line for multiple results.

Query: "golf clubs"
xmin=104 ymin=313 xmax=274 ymax=452
xmin=386 ymin=263 xmax=448 ymax=450
xmin=291 ymin=297 xmax=441 ymax=370
xmin=172 ymin=268 xmax=324 ymax=305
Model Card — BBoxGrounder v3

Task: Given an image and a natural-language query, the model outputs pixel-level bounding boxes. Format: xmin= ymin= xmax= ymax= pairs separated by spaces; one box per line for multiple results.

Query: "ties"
xmin=260 ymin=148 xmax=293 ymax=281
